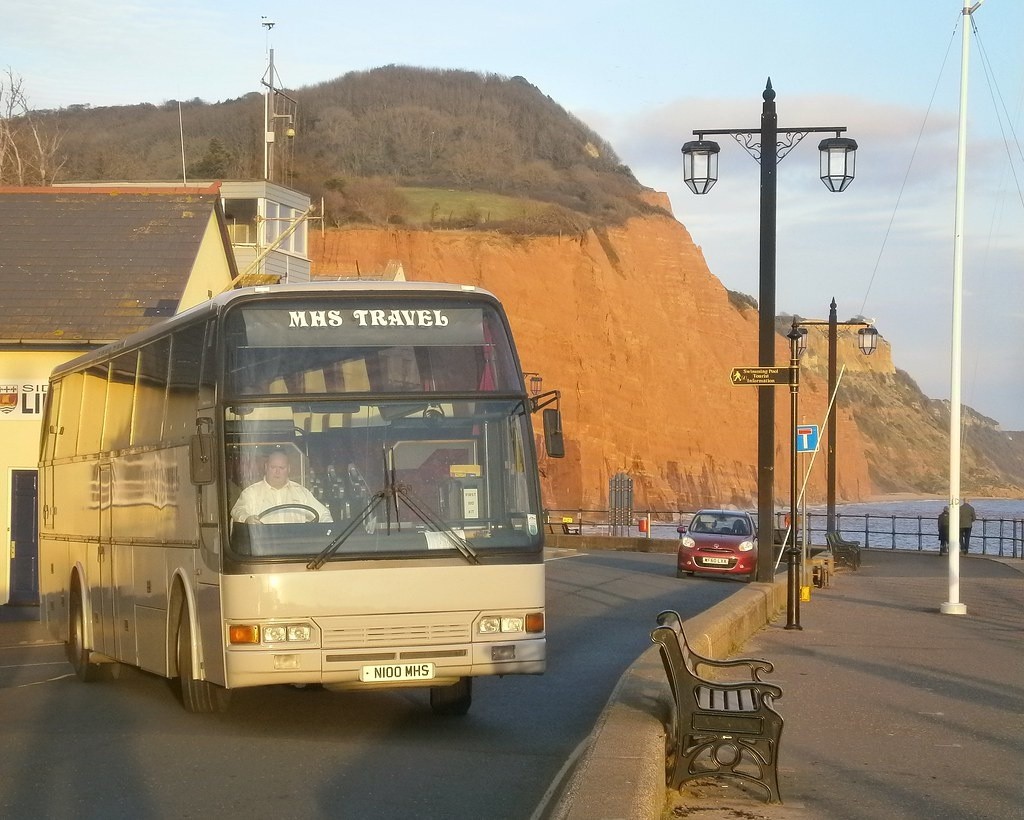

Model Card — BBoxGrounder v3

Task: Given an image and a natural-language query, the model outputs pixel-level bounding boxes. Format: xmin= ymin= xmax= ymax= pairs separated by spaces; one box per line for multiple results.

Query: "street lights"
xmin=680 ymin=77 xmax=861 ymax=588
xmin=788 ymin=295 xmax=879 ymax=548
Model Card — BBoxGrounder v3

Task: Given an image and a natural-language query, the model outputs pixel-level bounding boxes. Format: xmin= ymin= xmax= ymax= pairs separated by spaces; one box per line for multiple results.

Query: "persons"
xmin=230 ymin=452 xmax=334 ymax=523
xmin=938 ymin=497 xmax=977 ymax=556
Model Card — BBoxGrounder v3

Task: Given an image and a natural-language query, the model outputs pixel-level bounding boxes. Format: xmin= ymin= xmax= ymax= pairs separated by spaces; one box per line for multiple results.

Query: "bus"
xmin=35 ymin=276 xmax=566 ymax=725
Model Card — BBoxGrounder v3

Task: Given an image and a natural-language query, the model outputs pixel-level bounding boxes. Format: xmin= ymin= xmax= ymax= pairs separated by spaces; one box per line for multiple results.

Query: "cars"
xmin=676 ymin=509 xmax=759 ymax=583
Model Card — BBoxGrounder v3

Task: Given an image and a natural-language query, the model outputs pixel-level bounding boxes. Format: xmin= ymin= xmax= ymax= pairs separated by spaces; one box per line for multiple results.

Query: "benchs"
xmin=648 ymin=608 xmax=787 ymax=806
xmin=824 ymin=530 xmax=861 ymax=572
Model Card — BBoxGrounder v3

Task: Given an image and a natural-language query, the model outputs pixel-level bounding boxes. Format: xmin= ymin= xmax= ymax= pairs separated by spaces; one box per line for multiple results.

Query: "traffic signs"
xmin=730 ymin=365 xmax=800 ymax=387
